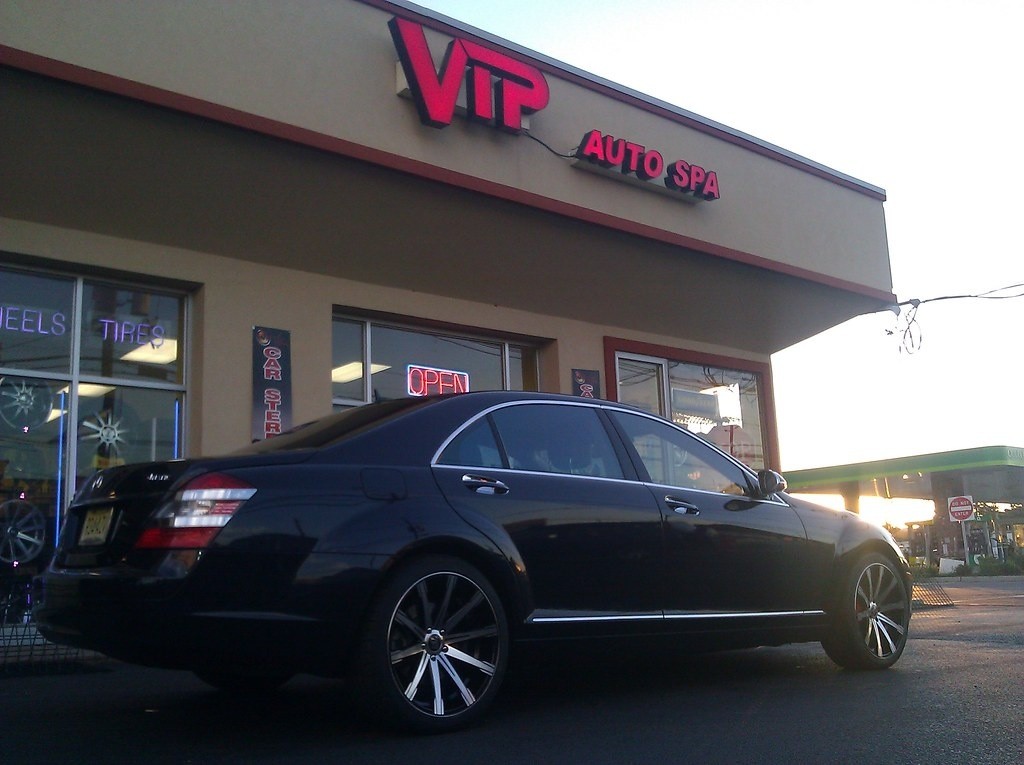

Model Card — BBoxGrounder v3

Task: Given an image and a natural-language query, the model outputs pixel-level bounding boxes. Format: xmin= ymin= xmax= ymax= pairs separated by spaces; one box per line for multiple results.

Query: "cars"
xmin=35 ymin=388 xmax=918 ymax=728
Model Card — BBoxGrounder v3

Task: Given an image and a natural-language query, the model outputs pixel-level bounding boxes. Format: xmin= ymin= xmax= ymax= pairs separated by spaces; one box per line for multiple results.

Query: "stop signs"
xmin=950 ymin=498 xmax=973 ymax=521
